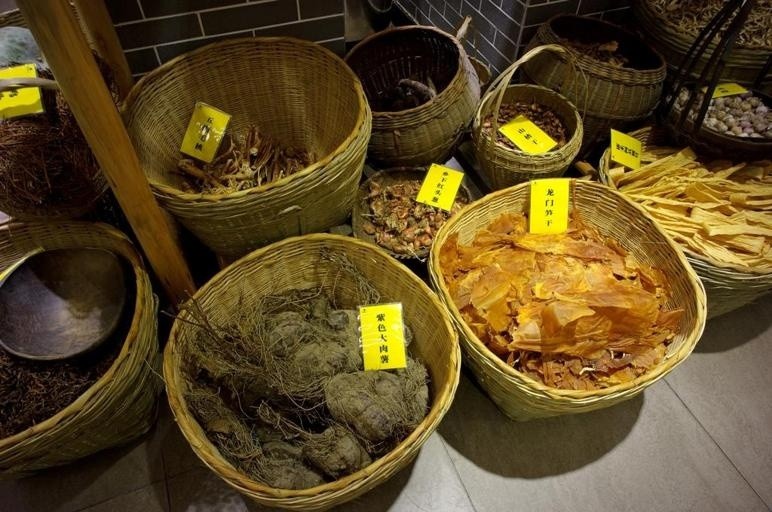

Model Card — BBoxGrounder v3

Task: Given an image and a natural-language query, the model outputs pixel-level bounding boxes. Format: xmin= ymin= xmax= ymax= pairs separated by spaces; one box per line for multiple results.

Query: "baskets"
xmin=0 ymin=2 xmax=768 ymax=512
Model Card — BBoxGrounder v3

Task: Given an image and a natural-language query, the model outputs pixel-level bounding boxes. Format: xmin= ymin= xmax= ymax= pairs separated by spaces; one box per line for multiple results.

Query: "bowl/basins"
xmin=1 ymin=243 xmax=126 ymax=362
